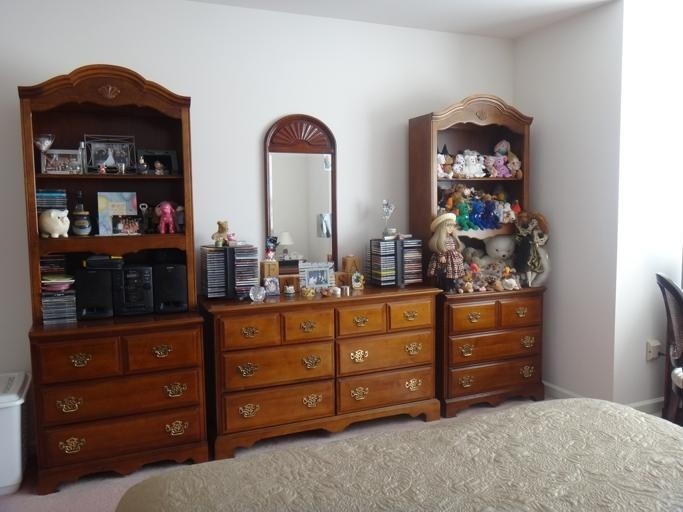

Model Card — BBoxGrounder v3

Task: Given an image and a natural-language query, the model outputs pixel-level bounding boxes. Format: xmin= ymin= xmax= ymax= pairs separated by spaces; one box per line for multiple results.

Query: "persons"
xmin=102 ymin=147 xmax=115 ymax=165
xmin=114 ymin=215 xmax=143 ymax=234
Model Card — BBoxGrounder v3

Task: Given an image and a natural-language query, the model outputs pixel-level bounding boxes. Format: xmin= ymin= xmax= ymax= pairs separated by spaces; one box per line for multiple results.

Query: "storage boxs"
xmin=0 ymin=370 xmax=33 ymax=496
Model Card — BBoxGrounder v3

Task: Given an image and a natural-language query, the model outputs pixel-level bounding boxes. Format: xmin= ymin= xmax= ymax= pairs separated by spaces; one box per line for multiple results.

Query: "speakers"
xmin=75 ymin=269 xmax=114 ymax=321
xmin=152 ymin=263 xmax=188 ymax=316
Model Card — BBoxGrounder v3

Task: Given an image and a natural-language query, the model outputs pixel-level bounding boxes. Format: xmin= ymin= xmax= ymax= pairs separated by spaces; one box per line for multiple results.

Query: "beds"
xmin=117 ymin=397 xmax=683 ymax=512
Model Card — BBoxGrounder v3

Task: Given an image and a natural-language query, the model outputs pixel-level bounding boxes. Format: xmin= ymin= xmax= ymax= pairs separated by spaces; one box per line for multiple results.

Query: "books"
xmin=35 ymin=188 xmax=66 ymax=213
xmin=39 ymin=253 xmax=77 ymax=326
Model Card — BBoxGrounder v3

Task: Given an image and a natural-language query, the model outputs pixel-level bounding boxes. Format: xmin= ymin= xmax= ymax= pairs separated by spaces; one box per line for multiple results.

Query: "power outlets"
xmin=646 ymin=339 xmax=661 ymax=360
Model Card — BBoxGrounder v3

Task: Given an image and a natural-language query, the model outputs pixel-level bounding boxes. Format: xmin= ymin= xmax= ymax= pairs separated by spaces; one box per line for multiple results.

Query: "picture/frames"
xmin=298 ymin=261 xmax=337 ymax=289
xmin=136 ymin=149 xmax=179 ymax=176
xmin=40 ymin=149 xmax=83 ymax=176
xmin=84 ymin=134 xmax=138 ymax=176
xmin=110 ymin=215 xmax=144 ymax=237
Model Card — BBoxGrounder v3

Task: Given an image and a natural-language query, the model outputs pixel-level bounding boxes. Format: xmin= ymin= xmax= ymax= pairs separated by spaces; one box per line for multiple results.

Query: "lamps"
xmin=278 ymin=231 xmax=295 ymax=254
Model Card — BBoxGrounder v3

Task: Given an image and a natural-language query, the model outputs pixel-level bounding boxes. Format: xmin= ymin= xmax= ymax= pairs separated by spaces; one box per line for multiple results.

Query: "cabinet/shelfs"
xmin=17 ymin=64 xmax=210 ymax=495
xmin=409 ymin=94 xmax=547 ymax=418
xmin=198 ymin=285 xmax=444 ymax=461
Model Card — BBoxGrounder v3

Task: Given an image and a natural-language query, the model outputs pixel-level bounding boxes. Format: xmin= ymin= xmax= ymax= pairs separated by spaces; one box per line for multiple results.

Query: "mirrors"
xmin=264 ymin=113 xmax=337 ymax=274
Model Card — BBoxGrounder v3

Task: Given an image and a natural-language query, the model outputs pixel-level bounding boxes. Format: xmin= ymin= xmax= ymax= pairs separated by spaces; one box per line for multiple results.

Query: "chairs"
xmin=656 ymin=274 xmax=683 ymax=425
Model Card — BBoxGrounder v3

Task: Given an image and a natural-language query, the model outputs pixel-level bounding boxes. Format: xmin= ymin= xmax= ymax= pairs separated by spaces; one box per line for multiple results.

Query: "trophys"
xmin=33 ymin=134 xmax=55 ymax=174
